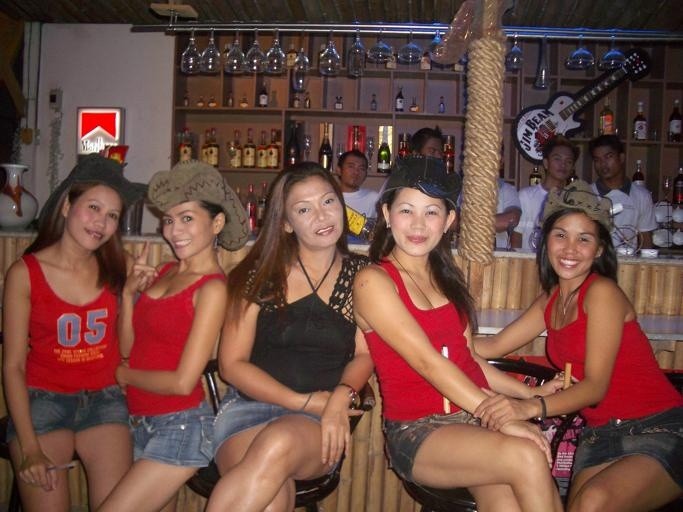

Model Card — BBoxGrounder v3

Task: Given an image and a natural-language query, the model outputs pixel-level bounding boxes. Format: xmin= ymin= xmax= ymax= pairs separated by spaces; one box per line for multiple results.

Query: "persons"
xmin=449 ymin=140 xmax=522 ymax=249
xmin=92 ymin=158 xmax=251 ymax=512
xmin=350 ymin=154 xmax=567 ymax=511
xmin=2 ymin=154 xmax=147 ymax=511
xmin=203 ymin=161 xmax=374 ymax=512
xmin=510 ymin=137 xmax=580 ymax=249
xmin=590 ymin=134 xmax=659 ymax=250
xmin=376 ymin=126 xmax=444 ymax=217
xmin=473 ymin=182 xmax=683 ymax=512
xmin=333 ymin=149 xmax=379 ymax=246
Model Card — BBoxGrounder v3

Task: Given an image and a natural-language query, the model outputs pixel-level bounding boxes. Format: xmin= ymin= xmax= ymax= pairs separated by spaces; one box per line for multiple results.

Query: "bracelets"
xmin=531 ymin=394 xmax=547 ymax=424
xmin=336 ymin=381 xmax=357 ymax=409
xmin=301 ymin=393 xmax=313 ymax=411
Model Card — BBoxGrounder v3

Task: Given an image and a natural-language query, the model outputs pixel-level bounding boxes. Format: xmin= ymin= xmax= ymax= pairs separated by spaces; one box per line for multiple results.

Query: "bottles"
xmin=285 ymin=121 xmax=453 ymax=173
xmin=597 ymin=97 xmax=682 ymax=142
xmin=672 ymin=168 xmax=683 ymax=204
xmin=529 ymin=166 xmax=541 ymax=186
xmin=179 ymin=126 xmax=282 ymax=170
xmin=177 ymin=80 xmax=461 ymax=113
xmin=631 ymin=160 xmax=644 ymax=187
xmin=566 ymin=170 xmax=579 ymax=186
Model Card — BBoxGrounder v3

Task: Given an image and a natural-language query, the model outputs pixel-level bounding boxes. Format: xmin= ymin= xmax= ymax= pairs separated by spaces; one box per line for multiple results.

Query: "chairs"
xmin=652 ymin=363 xmax=683 ymax=512
xmin=2 ymin=333 xmax=119 ymax=512
xmin=388 ymin=346 xmax=587 ymax=512
xmin=166 ymin=342 xmax=347 ymax=510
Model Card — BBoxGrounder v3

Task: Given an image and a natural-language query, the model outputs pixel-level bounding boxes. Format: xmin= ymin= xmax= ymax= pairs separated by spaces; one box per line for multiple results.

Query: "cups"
xmin=640 ymin=249 xmax=658 ymax=258
xmin=616 ymin=247 xmax=635 ymax=258
xmin=120 ymin=197 xmax=143 ymax=235
xmin=651 ymin=202 xmax=683 ymax=247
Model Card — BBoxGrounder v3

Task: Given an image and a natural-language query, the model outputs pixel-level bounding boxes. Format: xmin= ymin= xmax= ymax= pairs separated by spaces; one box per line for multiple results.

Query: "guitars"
xmin=510 ymin=50 xmax=652 ymax=164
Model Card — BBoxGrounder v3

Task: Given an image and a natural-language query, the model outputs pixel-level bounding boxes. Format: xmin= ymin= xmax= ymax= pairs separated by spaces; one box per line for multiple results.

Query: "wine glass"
xmin=180 ymin=19 xmax=469 ymax=77
xmin=662 ymin=175 xmax=671 ymax=204
xmin=504 ymin=33 xmax=625 ymax=88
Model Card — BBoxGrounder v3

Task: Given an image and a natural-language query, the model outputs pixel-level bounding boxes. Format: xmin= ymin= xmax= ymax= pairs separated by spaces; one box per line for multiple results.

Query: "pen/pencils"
xmin=564 ymin=362 xmax=572 ymax=389
xmin=440 ymin=344 xmax=452 ymax=414
xmin=48 ymin=465 xmax=75 ymax=471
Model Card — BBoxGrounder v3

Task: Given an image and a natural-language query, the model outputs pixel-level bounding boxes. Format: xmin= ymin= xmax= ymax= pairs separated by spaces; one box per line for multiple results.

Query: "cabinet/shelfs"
xmin=0 ymin=226 xmax=683 ymax=512
xmin=169 ymin=28 xmax=683 ymax=257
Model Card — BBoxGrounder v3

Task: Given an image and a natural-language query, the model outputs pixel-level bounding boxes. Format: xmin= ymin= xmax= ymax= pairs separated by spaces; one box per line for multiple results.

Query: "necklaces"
xmin=559 ymin=287 xmax=579 ymax=316
xmin=311 ymin=278 xmax=323 ymax=291
xmin=392 ymin=252 xmax=435 ymax=309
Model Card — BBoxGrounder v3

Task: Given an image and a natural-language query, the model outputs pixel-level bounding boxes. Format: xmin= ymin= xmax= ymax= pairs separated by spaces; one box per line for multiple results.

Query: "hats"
xmin=148 ymin=159 xmax=250 ymax=252
xmin=380 ymin=155 xmax=461 ymax=211
xmin=543 ymin=179 xmax=617 ymax=232
xmin=73 ymin=152 xmax=143 ymax=210
